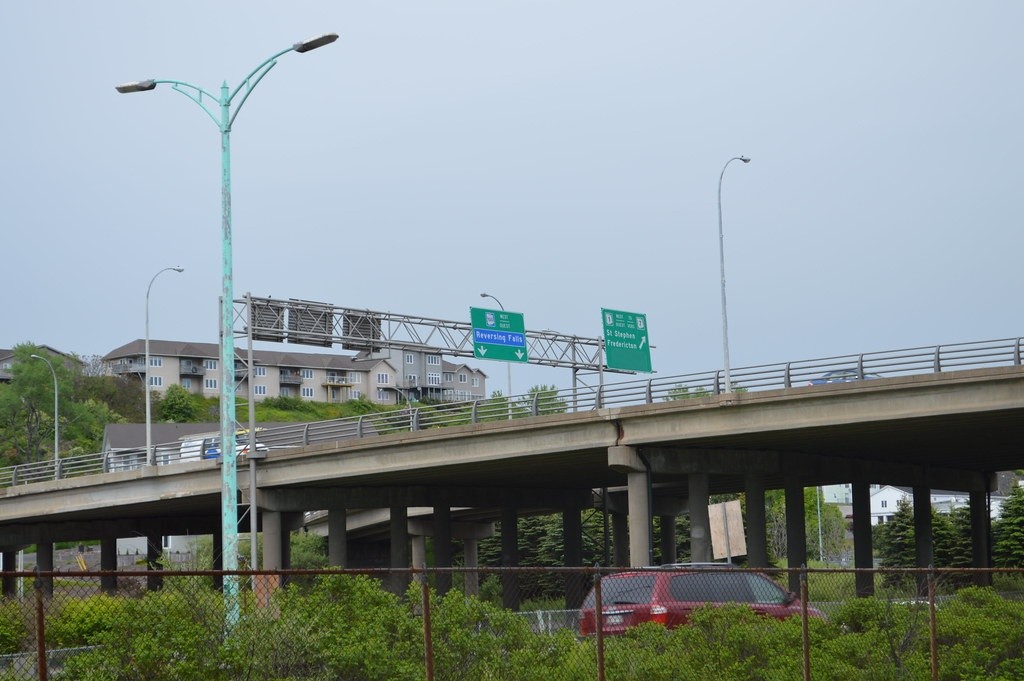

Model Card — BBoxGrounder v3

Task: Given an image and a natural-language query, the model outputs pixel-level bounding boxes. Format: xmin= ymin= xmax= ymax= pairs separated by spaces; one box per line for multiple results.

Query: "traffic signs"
xmin=602 ymin=308 xmax=653 ymax=373
xmin=469 ymin=306 xmax=527 ymax=362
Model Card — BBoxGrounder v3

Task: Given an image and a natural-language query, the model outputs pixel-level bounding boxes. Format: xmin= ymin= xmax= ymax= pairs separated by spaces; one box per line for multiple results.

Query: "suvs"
xmin=578 ymin=562 xmax=827 ymax=641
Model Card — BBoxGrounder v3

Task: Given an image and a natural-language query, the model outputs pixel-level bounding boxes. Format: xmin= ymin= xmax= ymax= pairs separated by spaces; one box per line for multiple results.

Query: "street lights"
xmin=482 ymin=293 xmax=513 ymax=420
xmin=145 ymin=268 xmax=185 ymax=466
xmin=718 ymin=156 xmax=752 ymax=394
xmin=31 ymin=354 xmax=60 ymax=481
xmin=115 ymin=33 xmax=337 ymax=626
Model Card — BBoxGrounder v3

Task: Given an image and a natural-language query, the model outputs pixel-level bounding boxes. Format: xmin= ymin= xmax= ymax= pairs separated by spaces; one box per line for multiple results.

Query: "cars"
xmin=808 ymin=368 xmax=882 ymax=386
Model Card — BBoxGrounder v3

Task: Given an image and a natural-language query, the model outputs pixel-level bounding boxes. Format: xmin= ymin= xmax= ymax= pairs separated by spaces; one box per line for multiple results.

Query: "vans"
xmin=179 ymin=434 xmax=270 ymax=464
